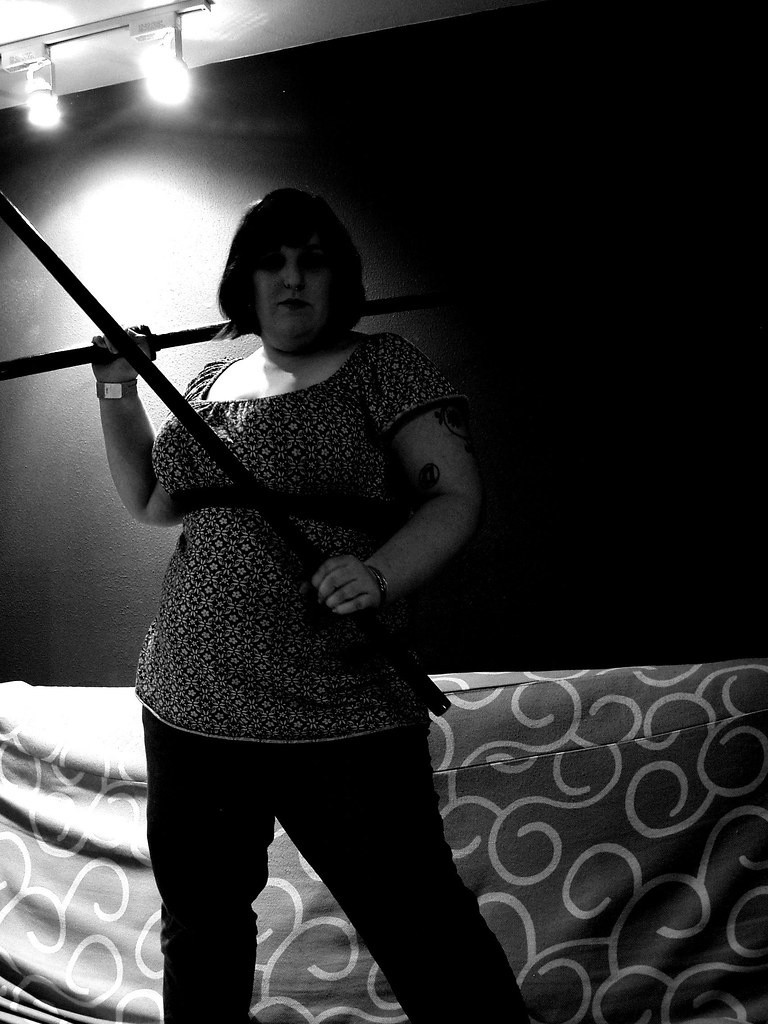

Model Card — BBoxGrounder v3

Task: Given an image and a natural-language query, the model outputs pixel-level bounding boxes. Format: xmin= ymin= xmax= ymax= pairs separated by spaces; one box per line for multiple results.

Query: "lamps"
xmin=129 ymin=11 xmax=191 ymax=106
xmin=2 ymin=42 xmax=61 ymax=128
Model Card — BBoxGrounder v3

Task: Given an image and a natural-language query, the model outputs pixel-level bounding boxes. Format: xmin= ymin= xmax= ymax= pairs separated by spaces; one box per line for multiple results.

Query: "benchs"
xmin=0 ymin=656 xmax=768 ymax=1024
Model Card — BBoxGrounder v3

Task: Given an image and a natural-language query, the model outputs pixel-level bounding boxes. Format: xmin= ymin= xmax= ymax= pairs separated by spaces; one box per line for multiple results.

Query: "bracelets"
xmin=366 ymin=564 xmax=388 ymax=609
xmin=96 ymin=379 xmax=137 ymax=400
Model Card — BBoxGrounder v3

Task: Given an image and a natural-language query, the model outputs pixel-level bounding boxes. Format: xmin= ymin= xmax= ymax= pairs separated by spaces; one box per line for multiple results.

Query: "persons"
xmin=93 ymin=187 xmax=531 ymax=1024
